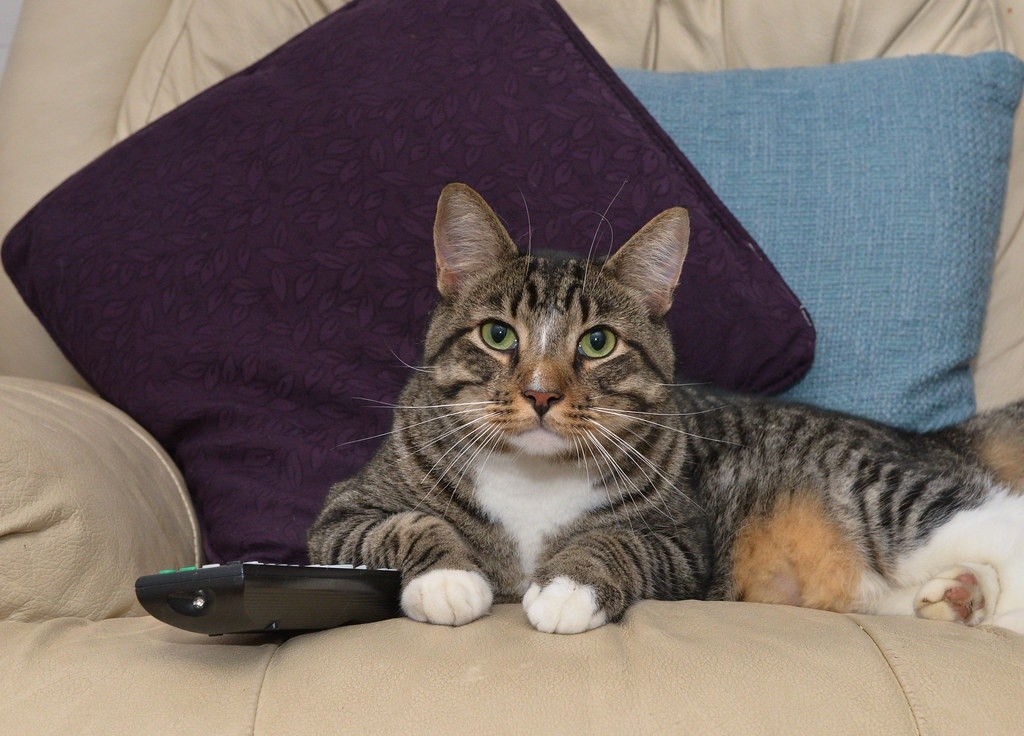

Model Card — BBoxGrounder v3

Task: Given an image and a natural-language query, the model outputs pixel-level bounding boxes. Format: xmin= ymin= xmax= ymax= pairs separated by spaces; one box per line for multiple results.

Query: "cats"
xmin=304 ymin=180 xmax=1022 ymax=638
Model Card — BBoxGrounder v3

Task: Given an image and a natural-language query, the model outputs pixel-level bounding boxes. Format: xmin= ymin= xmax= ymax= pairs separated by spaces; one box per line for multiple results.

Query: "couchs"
xmin=0 ymin=1 xmax=1024 ymax=735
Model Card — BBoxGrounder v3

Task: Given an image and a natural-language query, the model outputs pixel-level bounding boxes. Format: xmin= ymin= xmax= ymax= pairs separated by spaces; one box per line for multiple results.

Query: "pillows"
xmin=607 ymin=49 xmax=1023 ymax=437
xmin=0 ymin=0 xmax=816 ymax=567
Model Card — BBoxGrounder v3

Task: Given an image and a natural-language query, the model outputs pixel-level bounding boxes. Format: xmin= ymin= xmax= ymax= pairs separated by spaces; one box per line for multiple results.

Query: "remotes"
xmin=133 ymin=558 xmax=406 ymax=638
